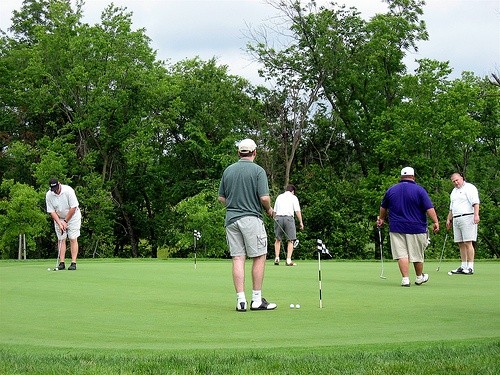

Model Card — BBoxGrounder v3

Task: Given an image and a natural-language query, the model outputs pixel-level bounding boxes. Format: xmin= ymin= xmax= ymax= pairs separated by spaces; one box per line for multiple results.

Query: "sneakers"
xmin=451 ymin=267 xmax=464 ymax=274
xmin=414 ymin=274 xmax=429 ymax=285
xmin=236 ymin=298 xmax=248 ymax=311
xmin=250 ymin=298 xmax=277 ymax=309
xmin=462 ymin=267 xmax=474 ymax=274
xmin=401 ymin=278 xmax=410 ymax=286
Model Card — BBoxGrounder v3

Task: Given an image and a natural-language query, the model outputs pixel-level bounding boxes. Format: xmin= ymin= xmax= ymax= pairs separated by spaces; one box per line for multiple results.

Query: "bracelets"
xmin=63 ymin=219 xmax=67 ymax=223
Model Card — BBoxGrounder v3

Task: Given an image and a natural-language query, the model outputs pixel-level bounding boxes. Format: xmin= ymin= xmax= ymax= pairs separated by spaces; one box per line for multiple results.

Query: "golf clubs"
xmin=436 ymin=227 xmax=449 ymax=271
xmin=272 ymin=216 xmax=300 ymax=248
xmin=377 ymin=216 xmax=387 ymax=280
xmin=54 ymin=229 xmax=63 ymax=271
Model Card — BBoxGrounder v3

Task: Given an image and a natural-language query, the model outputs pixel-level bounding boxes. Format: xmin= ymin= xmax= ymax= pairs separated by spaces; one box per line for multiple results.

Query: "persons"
xmin=446 ymin=172 xmax=479 ymax=275
xmin=274 ymin=185 xmax=304 ymax=266
xmin=376 ymin=167 xmax=440 ymax=287
xmin=45 ymin=178 xmax=82 ymax=269
xmin=218 ymin=138 xmax=277 ymax=312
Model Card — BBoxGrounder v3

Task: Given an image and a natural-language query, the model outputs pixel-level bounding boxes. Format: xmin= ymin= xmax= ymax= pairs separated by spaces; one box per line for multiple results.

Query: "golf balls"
xmin=56 ymin=268 xmax=58 ymax=270
xmin=48 ymin=268 xmax=50 ymax=270
xmin=296 ymin=304 xmax=301 ymax=309
xmin=290 ymin=304 xmax=294 ymax=308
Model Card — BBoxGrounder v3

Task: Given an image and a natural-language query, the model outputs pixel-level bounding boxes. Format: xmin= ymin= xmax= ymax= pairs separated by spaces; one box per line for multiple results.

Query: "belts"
xmin=453 ymin=213 xmax=473 ymax=218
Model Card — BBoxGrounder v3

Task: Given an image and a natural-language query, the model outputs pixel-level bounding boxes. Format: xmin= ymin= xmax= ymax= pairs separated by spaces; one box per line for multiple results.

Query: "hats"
xmin=49 ymin=179 xmax=59 ymax=190
xmin=401 ymin=167 xmax=415 ymax=176
xmin=239 ymin=138 xmax=257 ymax=153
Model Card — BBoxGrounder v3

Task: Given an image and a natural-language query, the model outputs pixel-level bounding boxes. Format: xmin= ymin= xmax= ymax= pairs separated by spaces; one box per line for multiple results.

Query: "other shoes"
xmin=68 ymin=262 xmax=76 ymax=270
xmin=286 ymin=261 xmax=295 ymax=266
xmin=274 ymin=258 xmax=278 ymax=265
xmin=54 ymin=262 xmax=65 ymax=270
